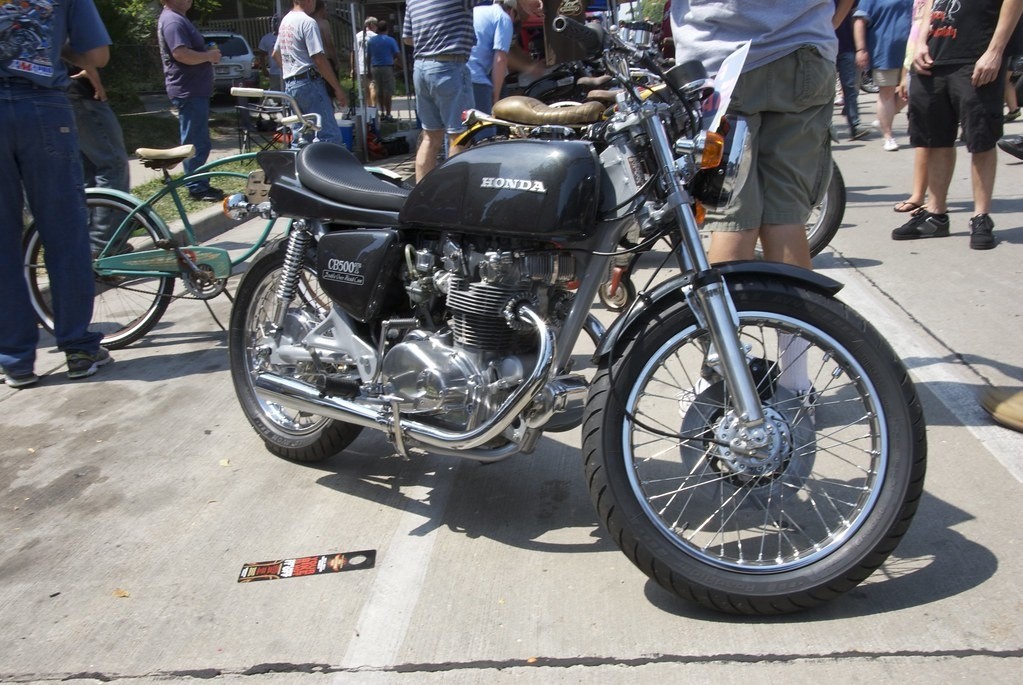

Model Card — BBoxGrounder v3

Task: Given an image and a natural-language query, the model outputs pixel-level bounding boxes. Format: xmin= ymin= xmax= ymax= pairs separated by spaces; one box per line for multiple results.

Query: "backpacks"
xmin=0 ymin=0 xmax=59 ymax=89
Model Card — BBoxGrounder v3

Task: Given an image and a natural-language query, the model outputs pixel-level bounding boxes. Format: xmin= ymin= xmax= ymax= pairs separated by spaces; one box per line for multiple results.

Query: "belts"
xmin=417 ymin=55 xmax=469 ymax=62
xmin=373 ymin=64 xmax=395 ymax=67
xmin=285 ymin=72 xmax=322 ymax=80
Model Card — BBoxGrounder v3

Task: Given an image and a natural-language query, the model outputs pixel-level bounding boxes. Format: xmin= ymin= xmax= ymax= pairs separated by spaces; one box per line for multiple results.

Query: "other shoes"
xmin=975 ymin=386 xmax=1023 ymax=433
xmin=884 ymin=138 xmax=900 ymax=150
xmin=93 ymin=242 xmax=133 ymax=283
xmin=998 ymin=135 xmax=1022 ymax=162
xmin=1002 ymin=108 xmax=1021 ymax=123
xmin=788 ymin=380 xmax=816 ymax=425
xmin=191 ymin=185 xmax=223 ymax=200
xmin=381 ymin=113 xmax=393 ymax=120
xmin=853 ymin=128 xmax=870 ymax=140
xmin=677 ymin=377 xmax=703 ymax=419
xmin=871 ymin=120 xmax=881 ymax=131
xmin=129 ymin=217 xmax=146 ymax=230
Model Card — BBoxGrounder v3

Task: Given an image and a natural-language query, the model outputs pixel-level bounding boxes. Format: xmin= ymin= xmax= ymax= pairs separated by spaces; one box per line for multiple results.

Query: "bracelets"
xmin=856 ymin=48 xmax=867 ymax=53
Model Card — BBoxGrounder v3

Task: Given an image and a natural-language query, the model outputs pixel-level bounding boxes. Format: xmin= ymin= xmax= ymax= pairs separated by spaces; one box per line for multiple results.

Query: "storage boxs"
xmin=339 ymin=118 xmax=354 ymax=153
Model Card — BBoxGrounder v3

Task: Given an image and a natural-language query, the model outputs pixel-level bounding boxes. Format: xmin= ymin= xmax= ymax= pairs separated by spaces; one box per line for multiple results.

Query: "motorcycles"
xmin=230 ymin=4 xmax=928 ymax=617
xmin=454 ymin=21 xmax=844 ymax=255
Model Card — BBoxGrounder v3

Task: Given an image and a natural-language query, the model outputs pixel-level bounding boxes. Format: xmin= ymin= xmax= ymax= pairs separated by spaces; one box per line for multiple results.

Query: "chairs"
xmin=231 ymin=76 xmax=292 ymax=167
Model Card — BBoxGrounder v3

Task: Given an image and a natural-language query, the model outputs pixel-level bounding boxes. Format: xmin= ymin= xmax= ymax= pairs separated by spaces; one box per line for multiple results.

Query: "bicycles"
xmin=21 ymin=87 xmax=321 ymax=349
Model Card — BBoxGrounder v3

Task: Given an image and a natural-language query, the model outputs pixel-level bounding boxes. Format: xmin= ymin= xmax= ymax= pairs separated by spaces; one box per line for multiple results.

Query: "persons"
xmin=469 ymin=0 xmax=1023 ymax=249
xmin=258 ymin=0 xmax=401 ymax=144
xmin=157 ymin=0 xmax=223 ymax=200
xmin=668 ymin=0 xmax=840 ymax=421
xmin=0 ymin=0 xmax=113 ymax=388
xmin=403 ymin=0 xmax=485 ymax=186
xmin=66 ymin=62 xmax=134 ymax=260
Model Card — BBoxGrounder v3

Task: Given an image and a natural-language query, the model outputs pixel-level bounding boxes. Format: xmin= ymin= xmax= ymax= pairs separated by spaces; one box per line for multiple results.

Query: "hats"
xmin=493 ymin=0 xmax=520 ymax=21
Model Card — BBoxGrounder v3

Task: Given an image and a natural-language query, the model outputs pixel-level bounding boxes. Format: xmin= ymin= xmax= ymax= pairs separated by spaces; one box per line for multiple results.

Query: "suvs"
xmin=200 ymin=32 xmax=259 ymax=100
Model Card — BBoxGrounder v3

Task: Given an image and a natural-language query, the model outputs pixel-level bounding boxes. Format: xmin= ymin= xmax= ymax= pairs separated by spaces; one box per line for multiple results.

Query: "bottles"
xmin=208 ymin=42 xmax=218 ymax=64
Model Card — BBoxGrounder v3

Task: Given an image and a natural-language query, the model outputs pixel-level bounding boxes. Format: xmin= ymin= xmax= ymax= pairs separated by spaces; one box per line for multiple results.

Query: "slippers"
xmin=893 ymin=201 xmax=925 ymax=216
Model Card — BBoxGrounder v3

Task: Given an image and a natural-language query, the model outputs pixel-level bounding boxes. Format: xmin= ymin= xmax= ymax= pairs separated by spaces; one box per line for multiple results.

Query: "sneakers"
xmin=65 ymin=344 xmax=111 ymax=379
xmin=0 ymin=366 xmax=39 ymax=387
xmin=892 ymin=210 xmax=950 ymax=240
xmin=970 ymin=214 xmax=994 ymax=250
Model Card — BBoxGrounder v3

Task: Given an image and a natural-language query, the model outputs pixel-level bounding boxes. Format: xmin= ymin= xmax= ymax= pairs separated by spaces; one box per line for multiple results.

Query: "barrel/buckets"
xmin=336 ymin=119 xmax=353 ymax=152
